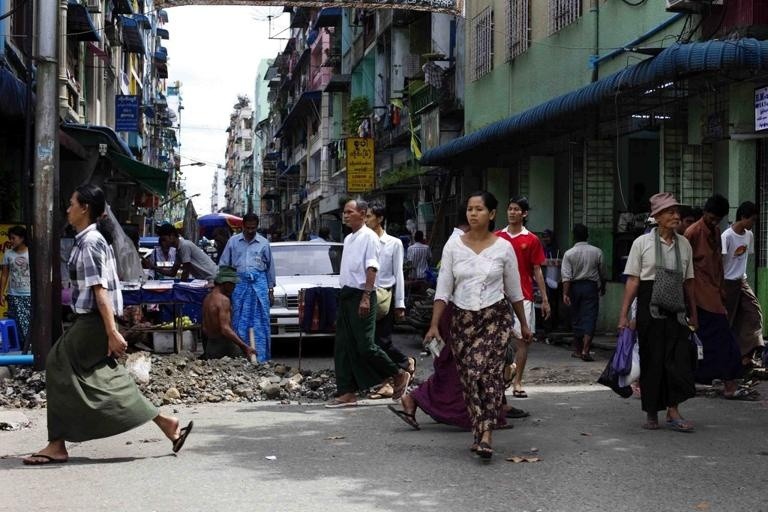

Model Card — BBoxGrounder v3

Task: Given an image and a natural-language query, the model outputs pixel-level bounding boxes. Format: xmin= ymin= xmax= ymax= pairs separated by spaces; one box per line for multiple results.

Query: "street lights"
xmin=152 ymin=161 xmax=205 ymax=236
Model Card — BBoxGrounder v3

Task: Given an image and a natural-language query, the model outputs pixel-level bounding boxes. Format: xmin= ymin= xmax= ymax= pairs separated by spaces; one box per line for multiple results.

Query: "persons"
xmin=388 ymin=191 xmax=550 ymax=457
xmin=617 ymin=192 xmax=766 ymax=431
xmin=202 ymin=212 xmax=277 ymax=367
xmin=309 ymin=198 xmax=416 ymax=408
xmin=150 ymin=223 xmax=218 ymax=282
xmin=561 ymin=224 xmax=607 ymax=362
xmin=0 ymin=183 xmax=192 ymax=466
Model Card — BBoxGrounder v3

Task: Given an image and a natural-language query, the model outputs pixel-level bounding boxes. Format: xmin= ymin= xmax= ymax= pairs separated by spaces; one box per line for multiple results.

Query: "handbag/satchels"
xmin=375 ymin=286 xmax=392 ymax=319
xmin=651 ymin=228 xmax=685 ymax=311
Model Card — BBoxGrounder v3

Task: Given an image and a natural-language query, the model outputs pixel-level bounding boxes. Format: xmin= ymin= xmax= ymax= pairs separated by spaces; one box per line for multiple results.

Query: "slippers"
xmin=173 ymin=421 xmax=193 ymax=452
xmin=368 ymin=357 xmax=416 ymax=401
xmin=325 ymin=399 xmax=356 ymax=407
xmin=666 ymin=419 xmax=696 ymax=432
xmin=502 ymin=373 xmax=529 ymax=418
xmin=727 ymin=386 xmax=758 ymax=400
xmin=388 ymin=404 xmax=420 ymax=430
xmin=642 ymin=417 xmax=658 ymax=429
xmin=476 ymin=443 xmax=492 ymax=458
xmin=23 ymin=453 xmax=67 ymax=464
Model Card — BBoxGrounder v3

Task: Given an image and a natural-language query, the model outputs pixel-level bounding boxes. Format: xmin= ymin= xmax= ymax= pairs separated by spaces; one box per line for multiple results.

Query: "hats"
xmin=650 ymin=192 xmax=690 ymax=217
xmin=214 ymin=267 xmax=240 ymax=284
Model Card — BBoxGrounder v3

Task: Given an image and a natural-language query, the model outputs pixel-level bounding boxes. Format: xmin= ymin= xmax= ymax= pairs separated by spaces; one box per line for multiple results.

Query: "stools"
xmin=0 ymin=318 xmax=21 ymax=353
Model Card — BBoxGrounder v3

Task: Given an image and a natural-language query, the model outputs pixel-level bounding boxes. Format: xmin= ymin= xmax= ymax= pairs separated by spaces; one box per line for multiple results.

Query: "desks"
xmin=118 ymin=289 xmax=210 ymax=355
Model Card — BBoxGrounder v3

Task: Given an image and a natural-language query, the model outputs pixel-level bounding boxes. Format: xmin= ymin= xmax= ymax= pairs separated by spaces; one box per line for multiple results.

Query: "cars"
xmin=268 ymin=241 xmax=349 ymax=339
